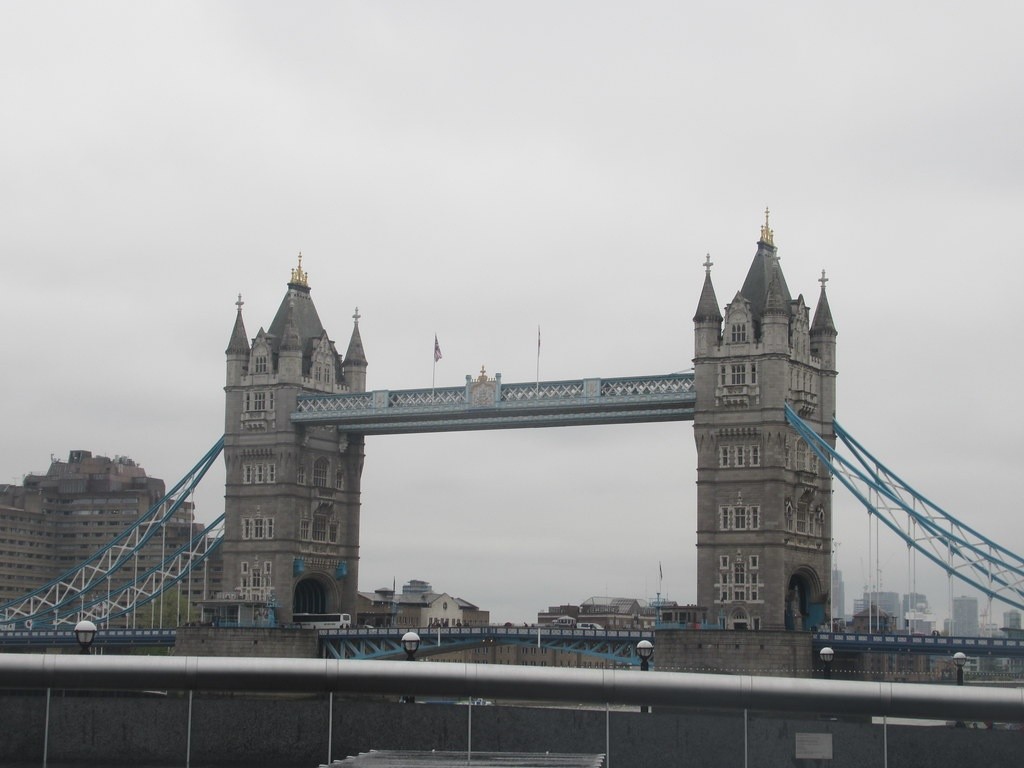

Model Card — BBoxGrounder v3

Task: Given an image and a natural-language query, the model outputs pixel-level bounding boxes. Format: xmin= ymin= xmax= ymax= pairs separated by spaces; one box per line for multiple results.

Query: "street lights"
xmin=636 ymin=640 xmax=654 ymax=713
xmin=953 ymin=652 xmax=967 ymax=727
xmin=819 ymin=647 xmax=835 ymax=716
xmin=402 ymin=631 xmax=421 ymax=702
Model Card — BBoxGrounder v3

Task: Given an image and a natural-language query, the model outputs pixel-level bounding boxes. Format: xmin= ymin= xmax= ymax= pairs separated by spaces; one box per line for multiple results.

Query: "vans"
xmin=577 ymin=623 xmax=604 ymax=630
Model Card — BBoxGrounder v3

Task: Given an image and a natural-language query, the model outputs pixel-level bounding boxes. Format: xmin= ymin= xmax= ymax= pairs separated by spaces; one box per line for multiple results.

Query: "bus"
xmin=293 ymin=613 xmax=351 ymax=629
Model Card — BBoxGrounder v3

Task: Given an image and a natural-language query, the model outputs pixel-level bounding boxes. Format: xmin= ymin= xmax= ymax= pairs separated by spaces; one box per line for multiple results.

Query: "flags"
xmin=434 ymin=335 xmax=442 ymax=362
